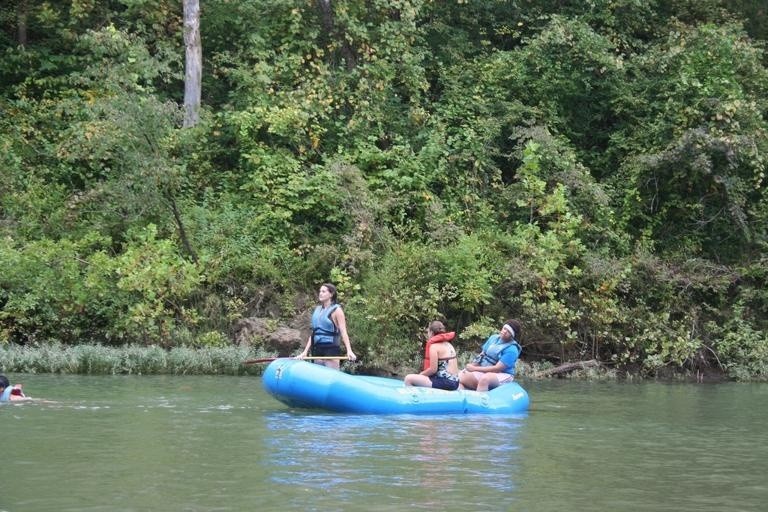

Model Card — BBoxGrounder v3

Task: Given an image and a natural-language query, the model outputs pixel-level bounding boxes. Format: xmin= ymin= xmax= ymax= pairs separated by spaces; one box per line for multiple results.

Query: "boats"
xmin=259 ymin=356 xmax=532 ymax=415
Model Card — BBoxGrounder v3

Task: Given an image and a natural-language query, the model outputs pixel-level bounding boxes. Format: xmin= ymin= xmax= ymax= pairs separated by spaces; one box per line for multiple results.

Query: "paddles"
xmin=244 ymin=356 xmax=350 ymax=364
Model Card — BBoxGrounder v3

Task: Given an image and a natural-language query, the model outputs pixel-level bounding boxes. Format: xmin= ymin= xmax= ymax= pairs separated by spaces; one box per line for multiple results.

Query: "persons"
xmin=296 ymin=283 xmax=357 ymax=371
xmin=0 ymin=375 xmax=31 ymax=402
xmin=456 ymin=319 xmax=521 ymax=393
xmin=404 ymin=319 xmax=460 ymax=391
